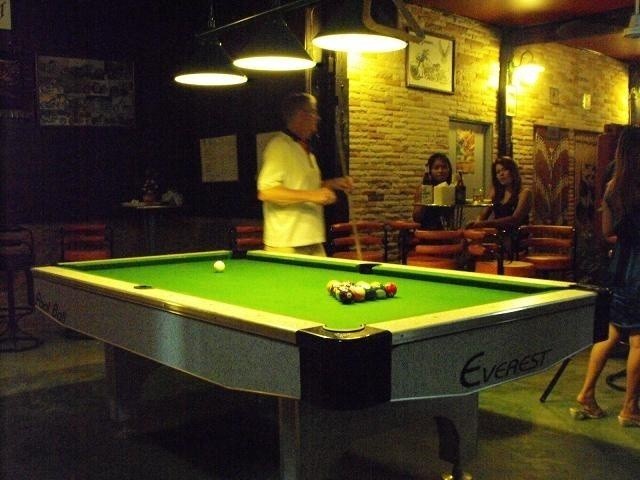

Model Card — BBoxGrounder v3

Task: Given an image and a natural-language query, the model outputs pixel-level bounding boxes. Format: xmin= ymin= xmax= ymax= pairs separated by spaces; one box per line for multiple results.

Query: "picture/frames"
xmin=31 ymin=49 xmax=139 ymax=131
xmin=405 ymin=27 xmax=455 ymax=95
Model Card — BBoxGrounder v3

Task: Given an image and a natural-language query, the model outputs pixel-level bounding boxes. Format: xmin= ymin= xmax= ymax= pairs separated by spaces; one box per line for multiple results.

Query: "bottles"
xmin=455 ymin=170 xmax=466 ymax=204
xmin=208 ymin=5 xmax=216 ymax=30
xmin=473 ymin=186 xmax=486 ymax=203
xmin=422 ymin=163 xmax=432 ymax=204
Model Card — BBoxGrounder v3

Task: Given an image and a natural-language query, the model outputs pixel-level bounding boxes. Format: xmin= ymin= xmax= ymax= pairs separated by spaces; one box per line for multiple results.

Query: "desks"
xmin=29 ymin=248 xmax=615 ymax=480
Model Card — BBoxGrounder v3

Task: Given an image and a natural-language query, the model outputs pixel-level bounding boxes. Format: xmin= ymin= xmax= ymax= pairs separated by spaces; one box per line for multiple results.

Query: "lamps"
xmin=170 ymin=1 xmax=408 ymax=89
xmin=511 ymin=47 xmax=545 ymax=85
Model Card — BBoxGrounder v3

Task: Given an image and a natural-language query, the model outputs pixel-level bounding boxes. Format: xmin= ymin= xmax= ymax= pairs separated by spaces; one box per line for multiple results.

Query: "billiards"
xmin=327 ymin=280 xmax=397 ymax=304
xmin=214 ymin=261 xmax=225 ymax=272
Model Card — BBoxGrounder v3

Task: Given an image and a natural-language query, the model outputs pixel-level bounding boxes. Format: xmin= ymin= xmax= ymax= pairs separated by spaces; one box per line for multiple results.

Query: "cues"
xmin=540 ymin=354 xmax=573 ymax=404
xmin=338 ymin=140 xmax=362 ymax=260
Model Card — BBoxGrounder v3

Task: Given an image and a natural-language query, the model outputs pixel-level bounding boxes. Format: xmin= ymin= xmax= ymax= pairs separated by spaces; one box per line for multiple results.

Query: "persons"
xmin=595 ymin=160 xmax=616 ymax=341
xmin=256 ymin=91 xmax=353 ymax=257
xmin=455 ymin=155 xmax=532 ymax=271
xmin=412 ymin=153 xmax=456 ymax=231
xmin=569 ymin=124 xmax=639 ymax=427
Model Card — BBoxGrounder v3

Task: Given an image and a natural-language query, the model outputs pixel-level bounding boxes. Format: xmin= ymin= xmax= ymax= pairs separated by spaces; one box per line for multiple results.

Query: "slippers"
xmin=618 ymin=415 xmax=640 ymax=428
xmin=569 ymin=407 xmax=607 ymax=420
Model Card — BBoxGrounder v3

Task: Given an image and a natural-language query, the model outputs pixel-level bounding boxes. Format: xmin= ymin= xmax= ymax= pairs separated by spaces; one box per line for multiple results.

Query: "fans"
xmin=556 ymin=0 xmax=640 ymax=48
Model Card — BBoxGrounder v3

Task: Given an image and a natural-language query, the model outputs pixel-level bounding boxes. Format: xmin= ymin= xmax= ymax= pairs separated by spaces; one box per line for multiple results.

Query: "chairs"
xmin=1 ymin=198 xmax=640 ymax=391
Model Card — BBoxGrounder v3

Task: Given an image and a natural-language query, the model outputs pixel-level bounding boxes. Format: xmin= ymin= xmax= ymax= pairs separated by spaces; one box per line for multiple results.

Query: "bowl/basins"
xmin=483 ymin=199 xmax=492 ymax=204
xmin=466 ymin=199 xmax=473 ymax=204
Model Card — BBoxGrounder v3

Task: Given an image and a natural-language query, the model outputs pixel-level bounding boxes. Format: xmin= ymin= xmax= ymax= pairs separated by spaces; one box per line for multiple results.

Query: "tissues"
xmin=433 ymin=181 xmax=450 ymax=205
